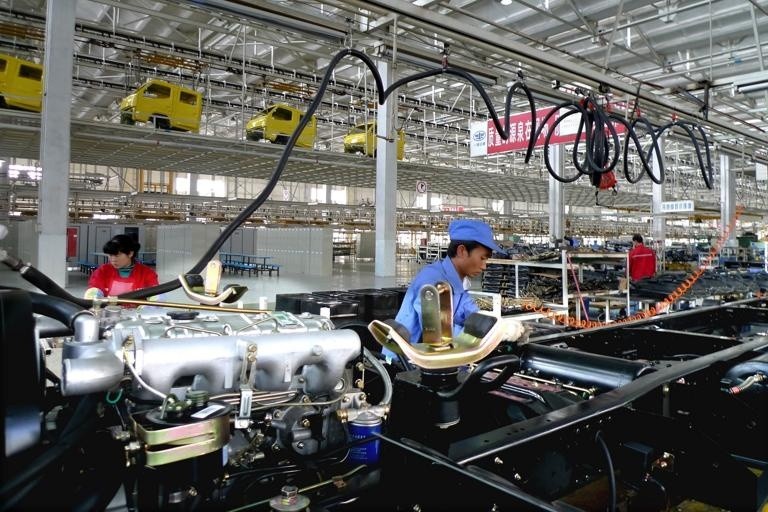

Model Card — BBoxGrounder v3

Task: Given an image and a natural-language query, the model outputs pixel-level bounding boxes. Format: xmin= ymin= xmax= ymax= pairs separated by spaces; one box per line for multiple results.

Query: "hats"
xmin=449 ymin=219 xmax=506 ymax=254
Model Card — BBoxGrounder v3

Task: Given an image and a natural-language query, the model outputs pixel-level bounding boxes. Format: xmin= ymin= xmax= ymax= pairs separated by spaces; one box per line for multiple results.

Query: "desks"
xmin=86 ymin=252 xmax=109 ymax=264
xmin=219 ymin=253 xmax=273 ymax=277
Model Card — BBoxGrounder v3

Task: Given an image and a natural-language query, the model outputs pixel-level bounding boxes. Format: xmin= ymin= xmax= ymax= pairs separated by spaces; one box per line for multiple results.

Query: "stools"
xmin=78 ymin=260 xmax=98 ymax=275
xmin=219 ymin=259 xmax=281 ymax=277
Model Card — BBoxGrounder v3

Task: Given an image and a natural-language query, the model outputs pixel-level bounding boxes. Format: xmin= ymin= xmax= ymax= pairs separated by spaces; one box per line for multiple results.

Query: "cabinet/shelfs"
xmin=482 ymin=247 xmax=630 ymax=325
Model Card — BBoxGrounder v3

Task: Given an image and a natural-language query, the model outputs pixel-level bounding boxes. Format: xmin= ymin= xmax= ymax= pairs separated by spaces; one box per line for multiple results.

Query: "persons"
xmin=380 ymin=218 xmax=509 ymax=366
xmin=84 ymin=234 xmax=160 ymax=310
xmin=626 ymin=235 xmax=656 ymax=282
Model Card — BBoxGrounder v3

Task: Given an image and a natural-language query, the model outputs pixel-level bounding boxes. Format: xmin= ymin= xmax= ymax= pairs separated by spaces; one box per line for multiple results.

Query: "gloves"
xmin=478 ymin=310 xmax=525 ymax=342
